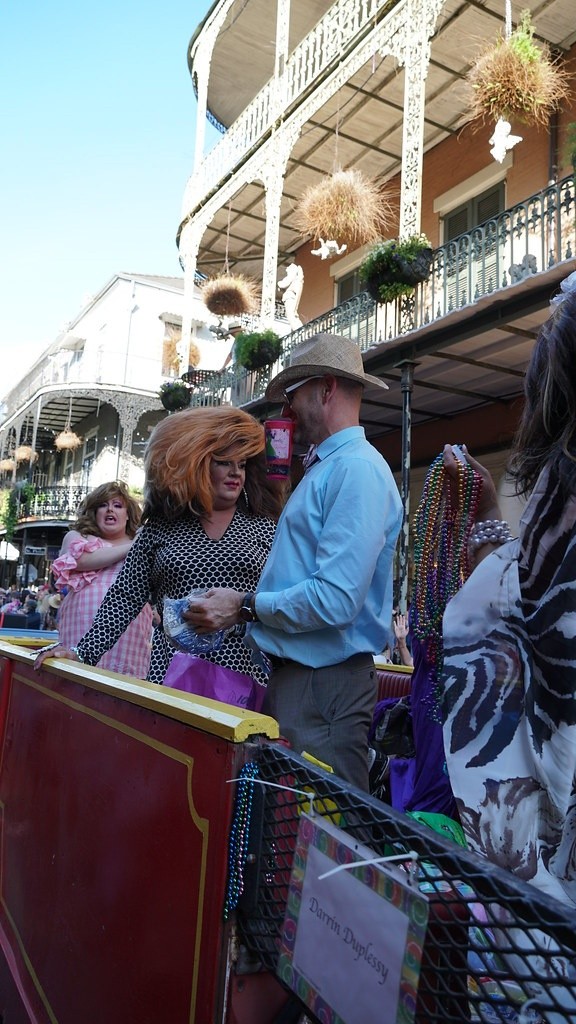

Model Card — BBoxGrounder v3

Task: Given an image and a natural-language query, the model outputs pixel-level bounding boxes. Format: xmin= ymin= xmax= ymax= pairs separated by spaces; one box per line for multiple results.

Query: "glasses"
xmin=281 ymin=374 xmax=326 ymax=406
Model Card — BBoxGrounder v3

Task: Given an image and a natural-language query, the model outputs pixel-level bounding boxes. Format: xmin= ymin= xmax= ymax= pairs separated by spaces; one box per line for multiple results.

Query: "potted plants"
xmin=233 ymin=328 xmax=283 ymax=370
xmin=0 ymin=482 xmax=47 ymax=544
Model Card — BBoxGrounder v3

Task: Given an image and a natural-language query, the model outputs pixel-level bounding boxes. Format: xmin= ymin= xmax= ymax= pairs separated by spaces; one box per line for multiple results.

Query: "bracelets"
xmin=468 ymin=520 xmax=513 ymax=555
xmin=70 ymin=647 xmax=85 ymax=663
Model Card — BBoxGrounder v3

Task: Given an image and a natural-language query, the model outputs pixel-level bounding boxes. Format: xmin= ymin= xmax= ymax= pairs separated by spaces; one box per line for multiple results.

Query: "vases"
xmin=162 ymin=388 xmax=190 ymax=411
xmin=367 ymin=247 xmax=435 ymax=303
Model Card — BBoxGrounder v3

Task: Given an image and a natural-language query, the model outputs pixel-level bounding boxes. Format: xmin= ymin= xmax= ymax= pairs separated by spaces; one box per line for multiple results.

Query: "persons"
xmin=278 ymin=263 xmax=305 ymax=330
xmin=180 ymin=335 xmax=404 ymax=858
xmin=51 ymin=482 xmax=154 ymax=681
xmin=407 ymin=272 xmax=576 ymax=1024
xmin=35 ymin=405 xmax=278 ymax=686
xmin=0 ymin=577 xmax=69 ymax=631
xmin=372 ymin=606 xmax=414 ymax=666
xmin=220 ymin=320 xmax=254 ymax=403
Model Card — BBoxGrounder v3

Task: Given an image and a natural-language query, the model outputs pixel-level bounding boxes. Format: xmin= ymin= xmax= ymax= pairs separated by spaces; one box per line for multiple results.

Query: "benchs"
xmin=376 ymin=663 xmax=415 ymax=705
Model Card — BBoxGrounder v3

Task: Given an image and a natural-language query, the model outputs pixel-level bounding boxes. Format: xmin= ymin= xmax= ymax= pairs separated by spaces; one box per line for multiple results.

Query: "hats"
xmin=264 ymin=333 xmax=390 ymax=402
xmin=48 ymin=595 xmax=61 ymax=608
xmin=57 ymin=588 xmax=67 ymax=595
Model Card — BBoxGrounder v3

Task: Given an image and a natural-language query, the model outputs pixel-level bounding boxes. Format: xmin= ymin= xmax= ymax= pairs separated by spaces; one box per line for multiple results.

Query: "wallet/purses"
xmin=164 ymin=597 xmax=231 ymax=654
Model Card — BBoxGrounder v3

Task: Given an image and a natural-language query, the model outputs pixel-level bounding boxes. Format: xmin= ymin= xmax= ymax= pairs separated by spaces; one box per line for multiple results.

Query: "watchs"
xmin=239 ymin=593 xmax=259 ymax=622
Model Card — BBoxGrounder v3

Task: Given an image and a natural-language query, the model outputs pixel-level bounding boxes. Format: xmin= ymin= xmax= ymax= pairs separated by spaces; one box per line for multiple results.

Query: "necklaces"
xmin=411 ymin=443 xmax=484 ymax=724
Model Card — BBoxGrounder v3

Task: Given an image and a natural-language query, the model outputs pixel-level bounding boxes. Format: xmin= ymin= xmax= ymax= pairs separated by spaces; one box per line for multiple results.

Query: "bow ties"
xmin=301 ymin=444 xmax=316 ymax=467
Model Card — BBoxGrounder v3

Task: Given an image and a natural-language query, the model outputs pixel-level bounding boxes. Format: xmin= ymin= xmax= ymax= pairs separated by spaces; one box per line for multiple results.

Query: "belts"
xmin=268 ymin=657 xmax=291 ymax=670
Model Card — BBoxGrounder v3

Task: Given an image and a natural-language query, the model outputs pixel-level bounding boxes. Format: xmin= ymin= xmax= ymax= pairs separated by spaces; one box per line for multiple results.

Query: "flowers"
xmin=157 ymin=380 xmax=186 ymax=398
xmin=354 ymin=233 xmax=433 ymax=304
